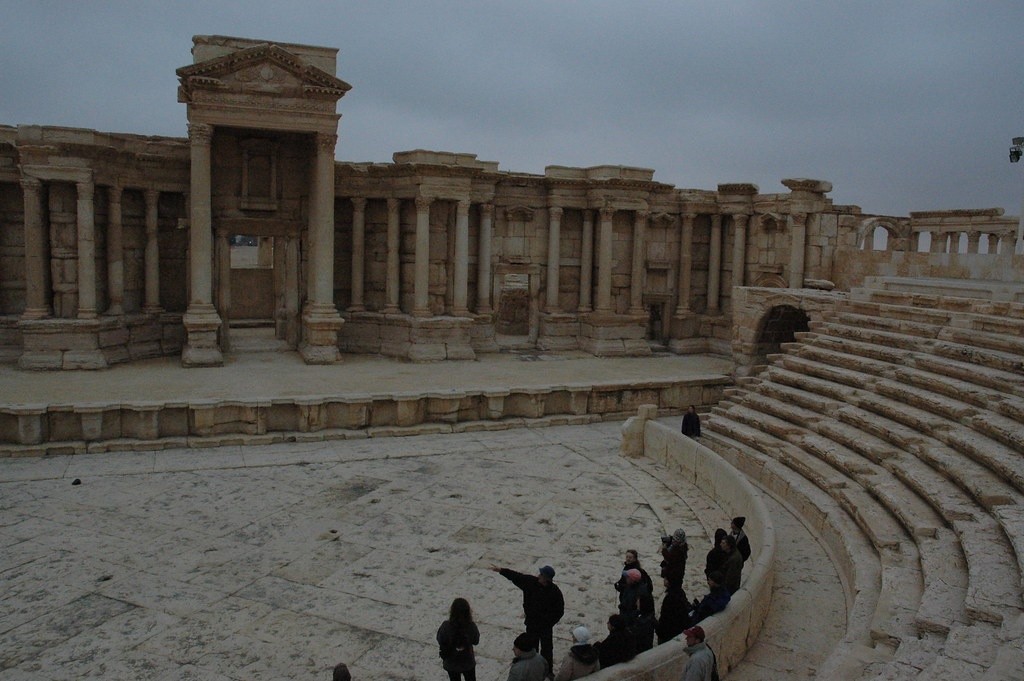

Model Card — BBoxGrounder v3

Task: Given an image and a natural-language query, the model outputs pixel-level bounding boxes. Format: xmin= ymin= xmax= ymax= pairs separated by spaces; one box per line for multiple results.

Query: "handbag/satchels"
xmin=712 ymin=671 xmax=720 ymax=681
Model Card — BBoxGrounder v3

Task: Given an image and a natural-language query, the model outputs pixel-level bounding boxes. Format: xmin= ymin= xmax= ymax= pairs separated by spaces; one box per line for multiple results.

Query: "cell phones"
xmin=694 ymin=599 xmax=699 ymax=604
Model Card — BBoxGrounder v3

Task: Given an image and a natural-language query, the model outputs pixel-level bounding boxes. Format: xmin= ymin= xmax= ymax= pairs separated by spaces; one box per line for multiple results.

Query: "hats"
xmin=514 ymin=632 xmax=536 ymax=651
xmin=733 ymin=517 xmax=745 ymax=529
xmin=573 ymin=626 xmax=591 ymax=642
xmin=539 ymin=565 xmax=555 ymax=578
xmin=674 ymin=529 xmax=685 ymax=543
xmin=622 ymin=569 xmax=641 ymax=582
xmin=609 ymin=614 xmax=627 ymax=628
xmin=683 ymin=625 xmax=705 ymax=640
xmin=710 ymin=572 xmax=723 ymax=584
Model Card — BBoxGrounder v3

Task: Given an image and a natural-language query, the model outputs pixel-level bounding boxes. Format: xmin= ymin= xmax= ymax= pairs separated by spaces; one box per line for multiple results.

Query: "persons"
xmin=491 ymin=564 xmax=565 ymax=676
xmin=333 ymin=663 xmax=351 ymax=681
xmin=601 ymin=528 xmax=689 ymax=668
xmin=436 ymin=598 xmax=480 ymax=681
xmin=681 ymin=626 xmax=717 ymax=681
xmin=682 ymin=405 xmax=701 ymax=438
xmin=507 ymin=632 xmax=549 ymax=681
xmin=706 ymin=517 xmax=751 ymax=595
xmin=554 ymin=627 xmax=600 ymax=681
xmin=688 ymin=573 xmax=732 ymax=622
